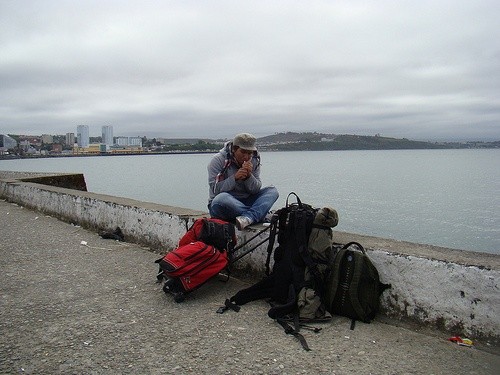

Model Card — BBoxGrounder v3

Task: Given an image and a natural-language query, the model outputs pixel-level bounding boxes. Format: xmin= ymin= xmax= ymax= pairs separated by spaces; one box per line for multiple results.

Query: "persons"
xmin=207 ymin=133 xmax=279 ymax=232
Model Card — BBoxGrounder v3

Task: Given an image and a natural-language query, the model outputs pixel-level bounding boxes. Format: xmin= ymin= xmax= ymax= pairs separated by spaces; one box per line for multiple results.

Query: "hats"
xmin=233 ymin=133 xmax=258 ymax=151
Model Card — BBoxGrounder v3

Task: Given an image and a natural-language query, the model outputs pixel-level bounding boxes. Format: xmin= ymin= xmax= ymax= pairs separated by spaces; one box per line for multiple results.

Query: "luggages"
xmin=154 ymin=218 xmax=290 ymax=303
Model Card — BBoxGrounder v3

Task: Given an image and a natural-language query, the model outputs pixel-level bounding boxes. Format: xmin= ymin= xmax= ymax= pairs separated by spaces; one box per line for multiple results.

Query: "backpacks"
xmin=216 ymin=192 xmax=321 ymax=321
xmin=177 ymin=215 xmax=238 ymax=253
xmin=323 ymin=241 xmax=391 ymax=330
